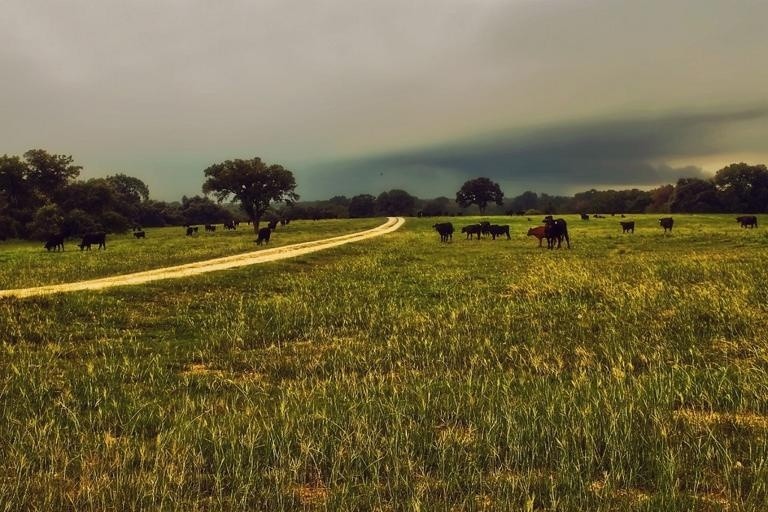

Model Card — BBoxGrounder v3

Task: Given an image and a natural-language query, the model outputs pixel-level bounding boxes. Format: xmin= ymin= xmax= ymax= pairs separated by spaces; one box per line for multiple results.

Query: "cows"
xmin=526 ymin=216 xmax=571 ymax=251
xmin=77 ymin=231 xmax=107 ymax=251
xmin=432 ymin=222 xmax=455 ymax=243
xmin=184 ymin=216 xmax=290 ymax=237
xmin=462 ymin=220 xmax=511 ymax=240
xmin=254 ymin=227 xmax=272 ymax=245
xmin=659 ymin=218 xmax=674 ymax=232
xmin=620 ymin=221 xmax=634 ymax=234
xmin=736 ymin=216 xmax=758 ymax=229
xmin=44 ymin=234 xmax=65 ymax=252
xmin=133 ymin=231 xmax=145 ymax=240
